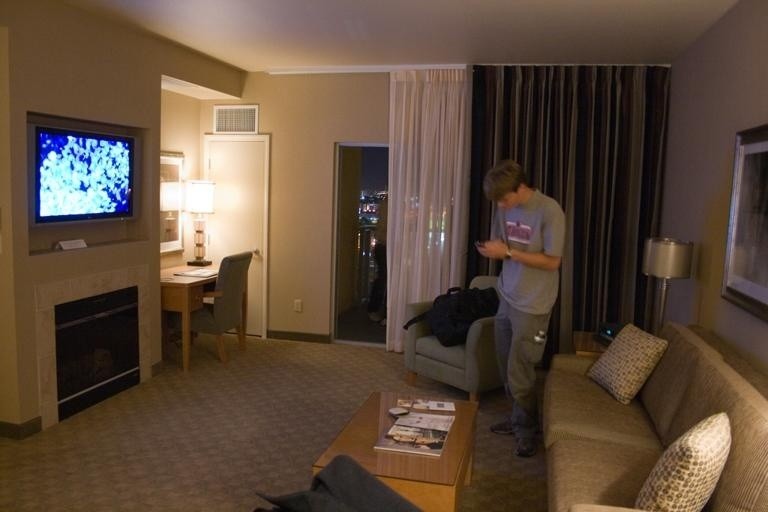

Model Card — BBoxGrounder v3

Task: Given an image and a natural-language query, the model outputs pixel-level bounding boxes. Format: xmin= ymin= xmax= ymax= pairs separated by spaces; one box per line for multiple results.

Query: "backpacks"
xmin=433 ymin=286 xmax=499 ymax=346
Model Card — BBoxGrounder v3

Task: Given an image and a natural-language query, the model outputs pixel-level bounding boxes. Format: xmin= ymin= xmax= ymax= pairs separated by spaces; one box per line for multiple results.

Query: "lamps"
xmin=157 ymin=181 xmax=179 ymax=243
xmin=180 ymin=179 xmax=215 ymax=270
xmin=643 ymin=234 xmax=693 ymax=336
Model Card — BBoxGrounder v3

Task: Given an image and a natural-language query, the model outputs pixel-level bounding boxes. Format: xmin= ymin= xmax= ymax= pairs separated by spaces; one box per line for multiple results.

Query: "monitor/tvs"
xmin=29 ymin=123 xmax=142 ymax=227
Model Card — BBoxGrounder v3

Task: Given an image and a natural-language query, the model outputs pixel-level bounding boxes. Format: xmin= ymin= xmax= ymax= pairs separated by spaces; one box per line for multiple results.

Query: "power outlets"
xmin=293 ymin=300 xmax=302 ymax=312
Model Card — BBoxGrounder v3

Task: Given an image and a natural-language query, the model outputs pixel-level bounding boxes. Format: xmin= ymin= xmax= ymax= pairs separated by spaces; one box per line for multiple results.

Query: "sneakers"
xmin=516 ymin=432 xmax=537 ymax=457
xmin=492 ymin=418 xmax=537 ymax=433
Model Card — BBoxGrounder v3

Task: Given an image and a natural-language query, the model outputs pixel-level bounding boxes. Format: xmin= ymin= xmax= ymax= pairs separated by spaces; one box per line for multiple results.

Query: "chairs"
xmin=168 ymin=250 xmax=254 ymax=370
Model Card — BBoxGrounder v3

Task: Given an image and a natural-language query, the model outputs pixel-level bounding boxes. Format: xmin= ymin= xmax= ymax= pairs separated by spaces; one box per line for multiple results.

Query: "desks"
xmin=312 ymin=388 xmax=481 ymax=512
xmin=569 ymin=325 xmax=614 ymax=353
xmin=158 ymin=263 xmax=249 ymax=371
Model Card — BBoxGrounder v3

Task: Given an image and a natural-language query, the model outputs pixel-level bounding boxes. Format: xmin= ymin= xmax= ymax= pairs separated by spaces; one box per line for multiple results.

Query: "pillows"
xmin=635 ymin=411 xmax=732 ymax=511
xmin=587 ymin=322 xmax=669 ymax=405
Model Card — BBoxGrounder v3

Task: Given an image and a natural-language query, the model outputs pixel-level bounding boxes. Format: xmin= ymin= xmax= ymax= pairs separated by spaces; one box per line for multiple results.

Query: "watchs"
xmin=504 ymin=247 xmax=512 ymax=260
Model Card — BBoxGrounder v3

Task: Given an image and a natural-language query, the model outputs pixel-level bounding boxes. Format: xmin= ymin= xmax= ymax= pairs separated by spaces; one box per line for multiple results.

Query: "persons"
xmin=476 ymin=160 xmax=566 ymax=458
xmin=367 ymin=195 xmax=387 ymax=325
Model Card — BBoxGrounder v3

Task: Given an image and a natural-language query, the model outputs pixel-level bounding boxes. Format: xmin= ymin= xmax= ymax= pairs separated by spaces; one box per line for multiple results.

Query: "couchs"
xmin=541 ymin=319 xmax=768 ymax=511
xmin=404 ymin=275 xmax=503 ymax=408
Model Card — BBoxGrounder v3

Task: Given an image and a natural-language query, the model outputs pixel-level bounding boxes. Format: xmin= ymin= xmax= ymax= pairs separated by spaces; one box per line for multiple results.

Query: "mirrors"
xmin=153 ymin=151 xmax=182 ymax=255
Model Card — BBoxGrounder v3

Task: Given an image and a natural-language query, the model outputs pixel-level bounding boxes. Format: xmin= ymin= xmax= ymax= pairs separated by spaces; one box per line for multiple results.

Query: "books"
xmin=174 ymin=267 xmax=219 ymax=278
xmin=373 ymin=411 xmax=455 ymax=457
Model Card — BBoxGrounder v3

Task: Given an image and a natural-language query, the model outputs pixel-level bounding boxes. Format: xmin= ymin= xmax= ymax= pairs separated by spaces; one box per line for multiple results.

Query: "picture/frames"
xmin=720 ymin=125 xmax=768 ymax=323
